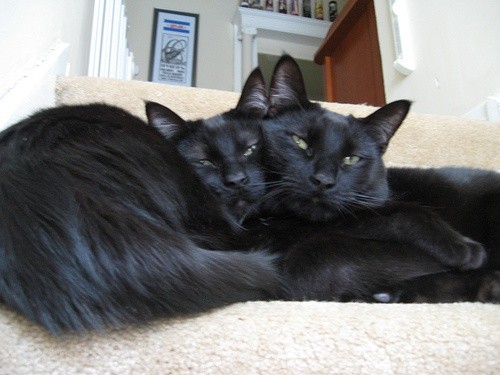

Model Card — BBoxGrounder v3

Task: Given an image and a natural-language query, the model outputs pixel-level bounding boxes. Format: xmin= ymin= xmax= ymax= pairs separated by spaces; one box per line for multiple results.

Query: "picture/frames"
xmin=148 ymin=5 xmax=200 ymax=88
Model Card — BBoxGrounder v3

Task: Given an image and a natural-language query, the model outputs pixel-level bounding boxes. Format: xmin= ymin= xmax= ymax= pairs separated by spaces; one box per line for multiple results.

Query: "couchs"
xmin=0 ymin=74 xmax=500 ymax=375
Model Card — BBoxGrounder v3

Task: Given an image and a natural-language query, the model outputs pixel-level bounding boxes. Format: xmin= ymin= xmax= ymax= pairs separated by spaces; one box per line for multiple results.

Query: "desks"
xmin=313 ymin=0 xmax=386 ymax=108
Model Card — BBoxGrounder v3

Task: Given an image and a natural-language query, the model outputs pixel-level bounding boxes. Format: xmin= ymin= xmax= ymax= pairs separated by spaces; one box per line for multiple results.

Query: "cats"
xmin=0 ymin=68 xmax=268 ymax=337
xmin=261 ymin=53 xmax=500 ymax=305
